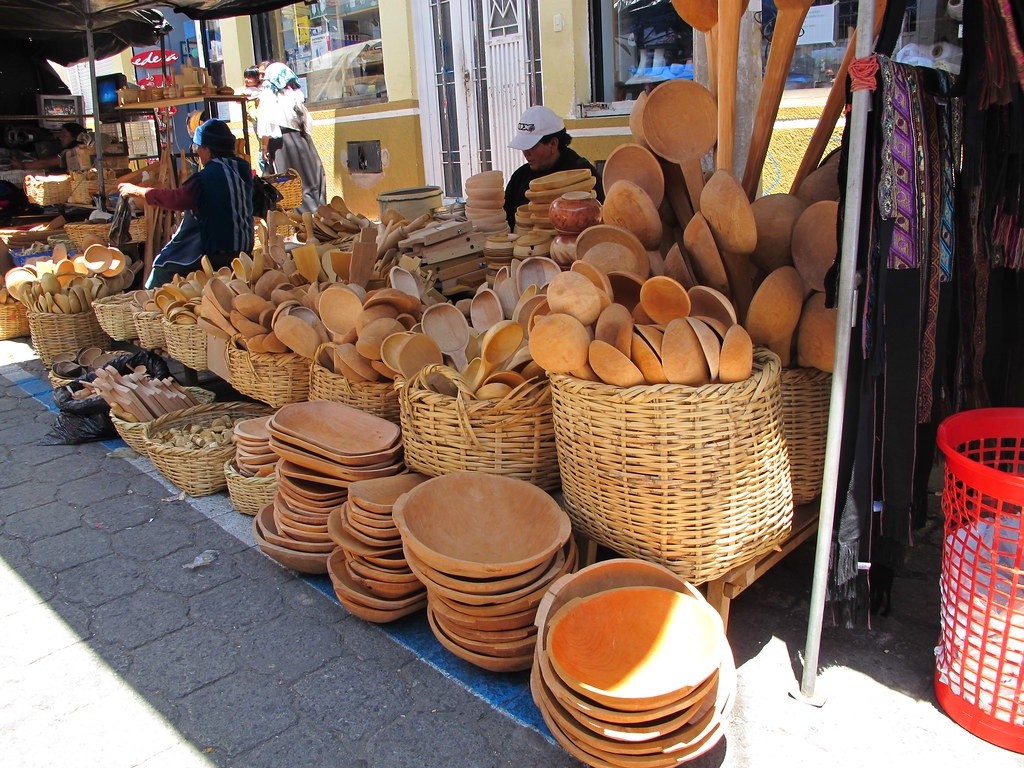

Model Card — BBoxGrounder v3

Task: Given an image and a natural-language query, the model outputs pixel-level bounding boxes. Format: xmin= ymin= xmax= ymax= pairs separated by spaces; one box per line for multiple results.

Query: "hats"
xmin=507 ymin=105 xmax=565 ymax=150
xmin=193 ymin=117 xmax=236 ymax=152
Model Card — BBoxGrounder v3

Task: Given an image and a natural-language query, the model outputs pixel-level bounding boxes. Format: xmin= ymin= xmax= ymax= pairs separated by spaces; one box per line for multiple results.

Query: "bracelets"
xmin=21 ymin=163 xmax=24 ymax=170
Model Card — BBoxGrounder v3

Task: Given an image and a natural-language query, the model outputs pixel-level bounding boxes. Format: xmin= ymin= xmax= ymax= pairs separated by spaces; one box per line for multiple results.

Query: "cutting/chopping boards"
xmin=92 ymin=365 xmax=201 ymax=423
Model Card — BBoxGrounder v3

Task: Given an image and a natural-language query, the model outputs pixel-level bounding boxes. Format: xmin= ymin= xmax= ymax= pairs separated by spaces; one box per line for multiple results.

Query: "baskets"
xmin=142 ymin=402 xmax=279 ymax=496
xmin=544 ymin=346 xmax=794 ymax=587
xmin=933 ymin=406 xmax=1024 ymax=754
xmin=48 ymin=351 xmax=132 ymax=391
xmin=90 ymin=290 xmax=155 ymax=341
xmin=133 ymin=312 xmax=167 ymax=350
xmin=8 ymin=248 xmax=76 ymax=267
xmin=0 ymin=302 xmax=30 ymax=340
xmin=276 ymin=208 xmax=294 ymax=235
xmin=25 ymin=308 xmax=112 ymax=369
xmin=225 ymin=334 xmax=312 ymax=407
xmin=392 ymin=364 xmax=560 ymax=492
xmin=308 ymin=341 xmax=401 ymax=432
xmin=70 ymin=179 xmax=117 ymax=204
xmin=108 ymin=386 xmax=217 ymax=459
xmin=162 ymin=317 xmax=209 ymax=372
xmin=25 ymin=175 xmax=71 ymax=206
xmin=0 ymin=222 xmax=65 ymax=248
xmin=64 ymin=216 xmax=147 ymax=247
xmin=224 ymin=458 xmax=277 ymax=516
xmin=48 ymin=234 xmax=77 ymax=252
xmin=779 ymin=367 xmax=833 ymax=506
xmin=261 ymin=168 xmax=303 ymax=210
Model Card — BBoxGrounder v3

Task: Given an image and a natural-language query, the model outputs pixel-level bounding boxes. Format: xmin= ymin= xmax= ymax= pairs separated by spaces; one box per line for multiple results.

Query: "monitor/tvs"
xmin=97 ymin=73 xmax=141 ymax=124
xmin=36 ymin=95 xmax=86 ymax=130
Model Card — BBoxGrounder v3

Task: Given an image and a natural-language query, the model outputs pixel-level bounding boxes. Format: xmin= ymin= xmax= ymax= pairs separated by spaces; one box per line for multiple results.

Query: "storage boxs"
xmin=309 ymin=32 xmax=339 ymax=59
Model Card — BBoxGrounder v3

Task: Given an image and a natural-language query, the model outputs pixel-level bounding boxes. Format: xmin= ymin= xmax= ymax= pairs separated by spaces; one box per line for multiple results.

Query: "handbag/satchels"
xmin=252 ymin=175 xmax=283 ymax=216
xmin=258 ymin=152 xmax=271 ymax=173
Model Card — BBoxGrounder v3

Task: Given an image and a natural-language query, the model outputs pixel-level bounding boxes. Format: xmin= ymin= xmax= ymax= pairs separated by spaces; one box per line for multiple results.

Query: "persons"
xmin=236 ymin=62 xmax=326 ymax=215
xmin=9 ymin=122 xmax=91 ymax=222
xmin=503 ymin=105 xmax=606 ymax=232
xmin=119 ymin=118 xmax=252 ymax=288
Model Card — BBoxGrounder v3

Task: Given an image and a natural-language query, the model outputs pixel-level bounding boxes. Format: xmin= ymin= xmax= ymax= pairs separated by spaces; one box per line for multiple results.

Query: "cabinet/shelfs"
xmin=275 ymin=0 xmax=382 ymax=77
xmin=115 ymin=94 xmax=250 ymax=188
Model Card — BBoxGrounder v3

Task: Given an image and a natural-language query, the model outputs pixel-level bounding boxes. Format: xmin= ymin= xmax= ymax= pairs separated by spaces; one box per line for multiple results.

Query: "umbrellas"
xmin=0 ymin=0 xmax=172 ymax=212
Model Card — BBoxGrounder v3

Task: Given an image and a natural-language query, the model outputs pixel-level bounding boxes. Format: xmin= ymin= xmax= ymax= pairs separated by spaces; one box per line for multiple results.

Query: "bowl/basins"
xmin=465 ymin=170 xmax=511 ymax=238
xmin=376 ymin=185 xmax=443 ymax=229
xmin=392 ymin=469 xmax=739 ymax=768
xmin=484 ymin=228 xmax=552 ymax=284
xmin=145 ymin=83 xmax=162 ymax=101
xmin=183 ymin=84 xmax=201 ymax=97
xmin=246 ymin=506 xmax=429 ymax=626
xmin=118 ymin=89 xmax=145 ymax=103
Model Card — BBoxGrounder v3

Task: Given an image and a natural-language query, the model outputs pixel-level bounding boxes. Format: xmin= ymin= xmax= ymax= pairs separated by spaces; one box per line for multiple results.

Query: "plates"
xmin=232 ymin=401 xmax=436 ymax=512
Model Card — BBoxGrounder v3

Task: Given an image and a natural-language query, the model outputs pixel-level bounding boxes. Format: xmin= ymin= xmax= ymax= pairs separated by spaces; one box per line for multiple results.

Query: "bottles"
xmin=160 ymin=57 xmax=217 ymax=98
